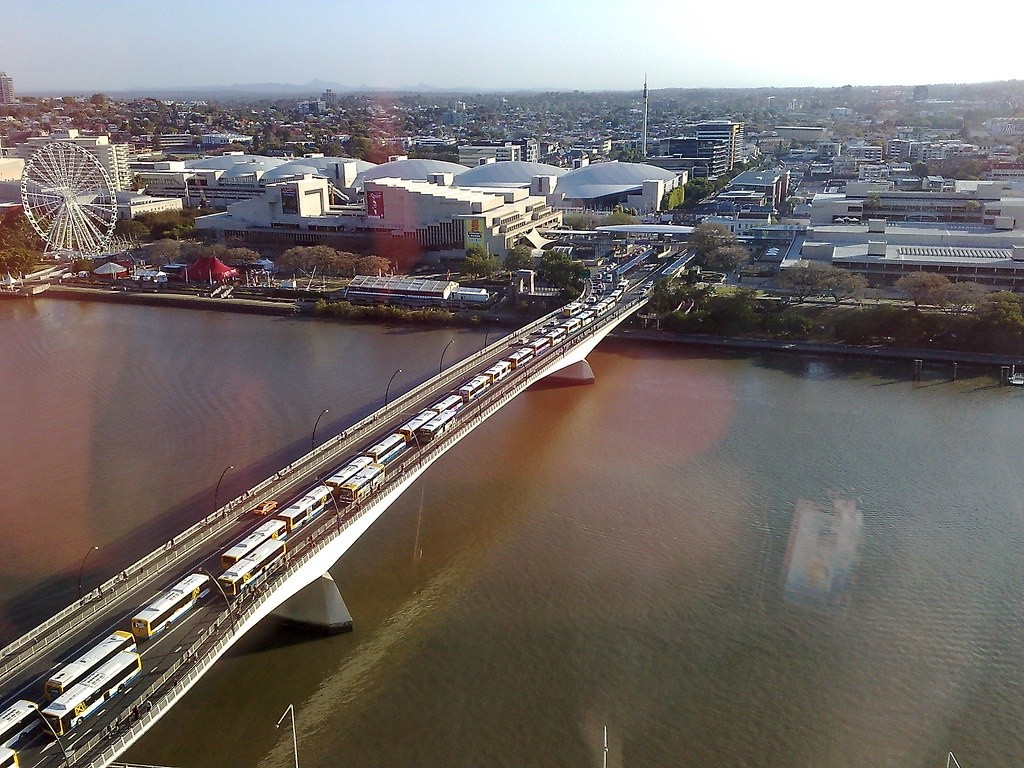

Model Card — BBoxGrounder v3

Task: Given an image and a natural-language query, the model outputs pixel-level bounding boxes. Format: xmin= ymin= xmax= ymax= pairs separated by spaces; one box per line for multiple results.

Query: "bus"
xmin=131 ymin=572 xmax=211 ymax=640
xmin=221 ymin=518 xmax=288 ymax=570
xmin=276 ymin=485 xmax=336 ymax=532
xmin=0 ymin=699 xmax=42 ymax=749
xmin=505 ymin=243 xmax=659 ymax=371
xmin=459 ymin=375 xmax=491 ymax=404
xmin=324 ymin=456 xmax=375 ymax=497
xmin=45 ymin=629 xmax=137 ymax=703
xmin=214 ymin=536 xmax=288 ymax=598
xmin=338 ymin=462 xmax=386 ymax=504
xmin=482 ymin=361 xmax=512 ymax=387
xmin=0 ymin=747 xmax=19 ymax=768
xmin=397 ymin=409 xmax=439 ymax=445
xmin=418 ymin=408 xmax=457 ymax=444
xmin=363 ymin=432 xmax=407 ymax=466
xmin=38 ymin=647 xmax=143 ymax=740
xmin=431 ymin=394 xmax=464 ymax=413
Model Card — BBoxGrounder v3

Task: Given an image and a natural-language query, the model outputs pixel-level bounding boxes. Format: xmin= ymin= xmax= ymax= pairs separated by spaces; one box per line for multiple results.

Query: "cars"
xmin=252 ymin=500 xmax=278 ymax=517
xmin=1008 ymin=372 xmax=1024 ymax=387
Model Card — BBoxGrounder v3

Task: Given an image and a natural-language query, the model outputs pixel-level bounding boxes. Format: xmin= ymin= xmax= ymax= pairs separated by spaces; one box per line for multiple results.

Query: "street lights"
xmin=214 ymin=466 xmax=234 ymax=514
xmin=485 ymin=319 xmax=498 ymax=348
xmin=78 ymin=546 xmax=99 ymax=598
xmin=440 ymin=340 xmax=454 ymax=373
xmin=385 ymin=369 xmax=402 ymax=407
xmin=313 ymin=409 xmax=329 ymax=450
